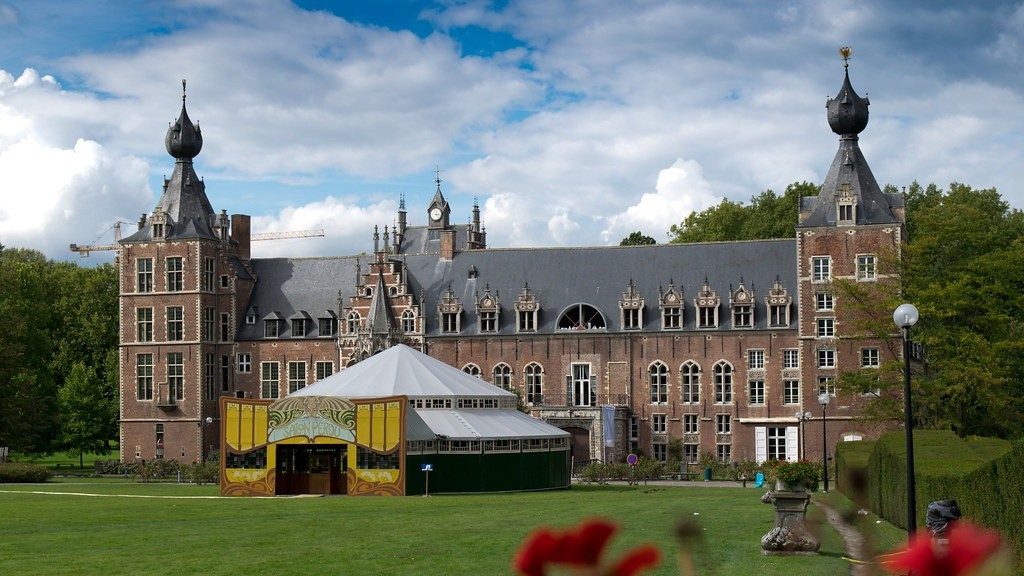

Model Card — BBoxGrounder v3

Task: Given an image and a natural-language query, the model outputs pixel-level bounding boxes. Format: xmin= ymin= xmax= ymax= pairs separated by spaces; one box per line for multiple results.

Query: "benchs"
xmin=667 ymin=472 xmax=700 ymax=480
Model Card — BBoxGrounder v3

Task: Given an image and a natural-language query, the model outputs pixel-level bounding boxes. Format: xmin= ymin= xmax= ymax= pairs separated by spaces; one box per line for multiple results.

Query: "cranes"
xmin=70 ymin=220 xmax=325 ymax=263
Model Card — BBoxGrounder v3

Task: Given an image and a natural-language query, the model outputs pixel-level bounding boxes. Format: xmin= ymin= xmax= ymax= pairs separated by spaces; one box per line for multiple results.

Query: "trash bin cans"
xmin=756 ymin=471 xmax=765 ymax=488
xmin=705 ymin=468 xmax=712 ymax=481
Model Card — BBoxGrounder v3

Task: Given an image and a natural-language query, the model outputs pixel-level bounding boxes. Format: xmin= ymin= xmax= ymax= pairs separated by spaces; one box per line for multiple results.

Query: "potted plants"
xmin=762 ymin=458 xmax=823 ymax=492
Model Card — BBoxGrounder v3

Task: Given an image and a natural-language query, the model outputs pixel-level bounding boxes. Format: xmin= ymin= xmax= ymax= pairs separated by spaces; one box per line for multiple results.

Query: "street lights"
xmin=202 ymin=417 xmax=212 ymax=465
xmin=818 ymin=393 xmax=831 ymax=492
xmin=794 ymin=412 xmax=812 ymax=461
xmin=893 ymin=302 xmax=918 ymax=552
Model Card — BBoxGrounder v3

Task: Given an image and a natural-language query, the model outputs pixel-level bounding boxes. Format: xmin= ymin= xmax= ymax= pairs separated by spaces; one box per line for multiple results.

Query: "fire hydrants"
xmin=924 ymin=499 xmax=961 ymax=553
